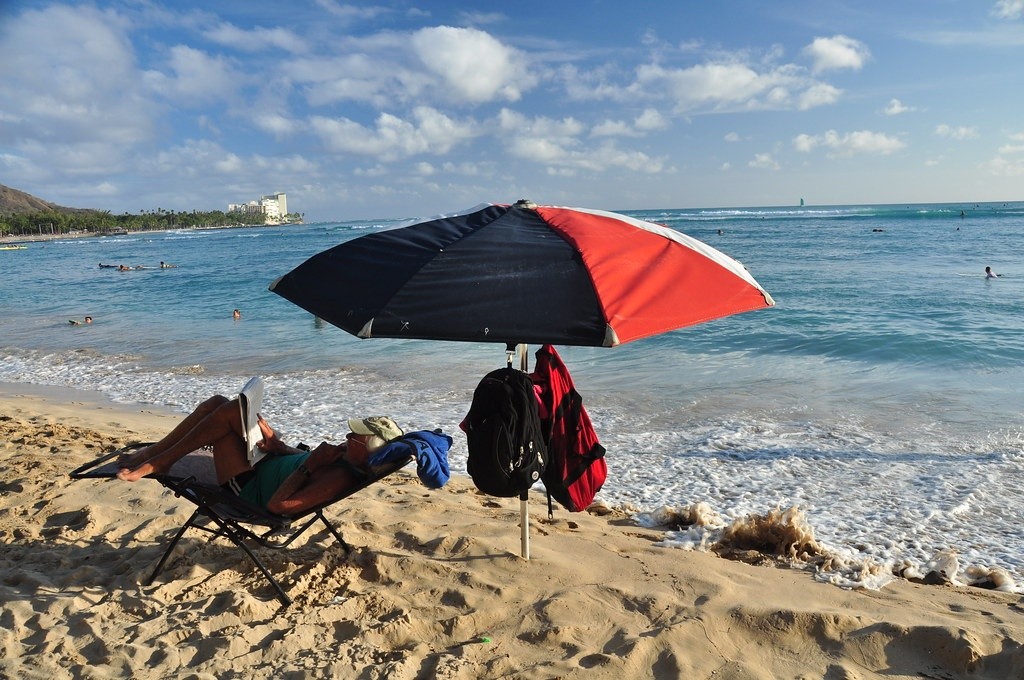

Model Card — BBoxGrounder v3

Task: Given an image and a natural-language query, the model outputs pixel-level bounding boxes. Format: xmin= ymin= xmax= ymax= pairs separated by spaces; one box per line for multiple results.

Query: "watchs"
xmin=298 ymin=464 xmax=312 ymax=478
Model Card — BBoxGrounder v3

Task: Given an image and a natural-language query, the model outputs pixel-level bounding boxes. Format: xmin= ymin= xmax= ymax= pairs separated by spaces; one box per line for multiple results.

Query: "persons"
xmin=83 ymin=260 xmax=240 ymax=324
xmin=984 ymin=266 xmax=1000 ymax=279
xmin=116 ymin=394 xmax=404 ymax=519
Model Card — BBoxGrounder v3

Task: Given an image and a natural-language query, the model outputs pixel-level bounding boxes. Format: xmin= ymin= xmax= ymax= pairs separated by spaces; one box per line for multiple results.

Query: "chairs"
xmin=68 ymin=429 xmax=453 ymax=609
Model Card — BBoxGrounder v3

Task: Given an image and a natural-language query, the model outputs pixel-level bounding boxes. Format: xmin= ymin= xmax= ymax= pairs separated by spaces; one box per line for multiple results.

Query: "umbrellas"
xmin=267 ymin=199 xmax=775 ymax=560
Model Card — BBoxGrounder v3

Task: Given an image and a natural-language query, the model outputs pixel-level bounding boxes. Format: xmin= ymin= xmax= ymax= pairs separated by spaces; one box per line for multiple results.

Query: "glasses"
xmin=350 ymin=432 xmax=368 ymax=448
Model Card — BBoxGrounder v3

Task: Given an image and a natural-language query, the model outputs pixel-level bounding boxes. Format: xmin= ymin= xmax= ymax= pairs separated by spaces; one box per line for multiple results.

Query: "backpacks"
xmin=467 ymin=363 xmax=548 ymax=497
xmin=527 ymin=344 xmax=607 ymax=513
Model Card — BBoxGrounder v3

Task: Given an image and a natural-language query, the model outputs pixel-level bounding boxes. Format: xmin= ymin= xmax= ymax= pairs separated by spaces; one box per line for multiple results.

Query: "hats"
xmin=347 ymin=415 xmax=404 ymax=444
